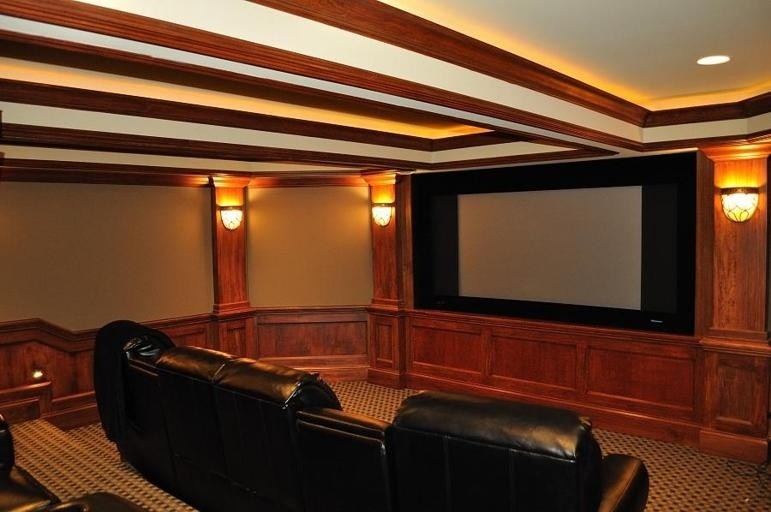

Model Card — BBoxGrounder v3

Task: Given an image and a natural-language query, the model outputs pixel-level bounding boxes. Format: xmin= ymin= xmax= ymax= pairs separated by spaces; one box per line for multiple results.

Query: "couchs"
xmin=0 ymin=414 xmax=145 ymax=512
xmin=93 ymin=320 xmax=649 ymax=510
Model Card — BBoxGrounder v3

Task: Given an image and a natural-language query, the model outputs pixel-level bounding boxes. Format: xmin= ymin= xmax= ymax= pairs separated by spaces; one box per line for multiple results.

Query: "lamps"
xmin=371 ymin=203 xmax=392 ymax=226
xmin=219 ymin=206 xmax=244 ymax=230
xmin=720 ymin=187 xmax=759 ymax=223
